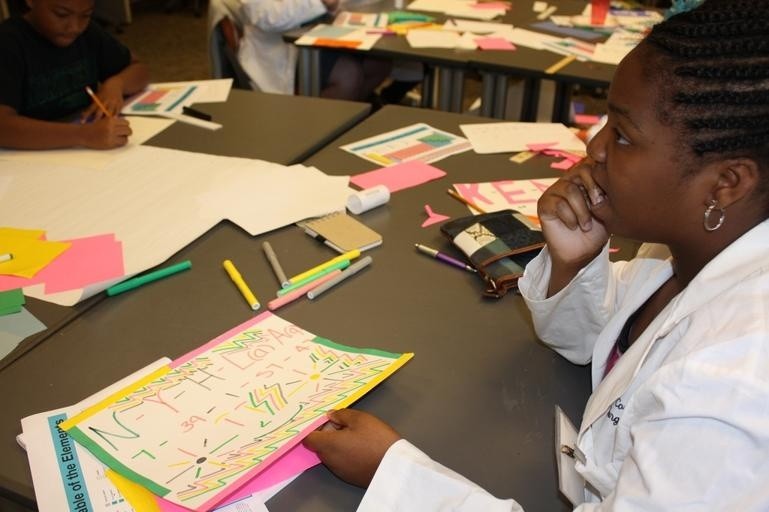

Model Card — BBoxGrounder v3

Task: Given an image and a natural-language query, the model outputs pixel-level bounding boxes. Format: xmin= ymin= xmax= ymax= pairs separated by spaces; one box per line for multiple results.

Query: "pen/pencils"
xmin=263 ymin=241 xmax=289 ymax=288
xmin=447 ymin=188 xmax=486 ymax=214
xmin=0 ymin=254 xmax=12 ymax=263
xmin=288 ymin=248 xmax=360 ymax=283
xmin=415 ymin=244 xmax=477 ymax=274
xmin=365 ymin=30 xmax=397 ymax=35
xmin=268 ymin=268 xmax=342 ymax=310
xmin=223 ymin=259 xmax=259 ymax=310
xmin=85 ymin=86 xmax=112 ymax=117
xmin=106 ymin=260 xmax=192 ymax=295
xmin=545 ymin=55 xmax=575 ymax=75
xmin=307 ymin=255 xmax=373 ymax=299
xmin=183 ymin=106 xmax=212 ymax=121
xmin=277 ymin=258 xmax=351 ymax=296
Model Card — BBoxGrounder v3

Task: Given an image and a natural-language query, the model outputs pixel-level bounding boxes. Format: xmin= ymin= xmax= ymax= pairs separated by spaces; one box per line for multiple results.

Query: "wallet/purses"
xmin=441 ymin=209 xmax=545 ymax=298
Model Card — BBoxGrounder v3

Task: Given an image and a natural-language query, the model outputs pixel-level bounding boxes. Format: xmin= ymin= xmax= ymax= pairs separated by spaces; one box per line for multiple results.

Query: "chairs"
xmin=220 ymin=13 xmax=252 ymax=89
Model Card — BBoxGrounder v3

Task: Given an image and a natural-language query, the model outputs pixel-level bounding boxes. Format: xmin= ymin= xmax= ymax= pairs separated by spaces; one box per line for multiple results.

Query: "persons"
xmin=298 ymin=0 xmax=767 ymax=512
xmin=206 ymin=0 xmax=390 ymax=101
xmin=0 ymin=0 xmax=149 ymax=149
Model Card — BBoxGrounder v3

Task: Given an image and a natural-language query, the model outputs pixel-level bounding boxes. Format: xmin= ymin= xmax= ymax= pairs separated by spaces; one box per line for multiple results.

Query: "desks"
xmin=0 ymin=89 xmax=372 ymax=372
xmin=470 ymin=0 xmax=671 ymax=123
xmin=0 ymin=101 xmax=645 ymax=512
xmin=281 ymin=0 xmax=559 ymax=114
